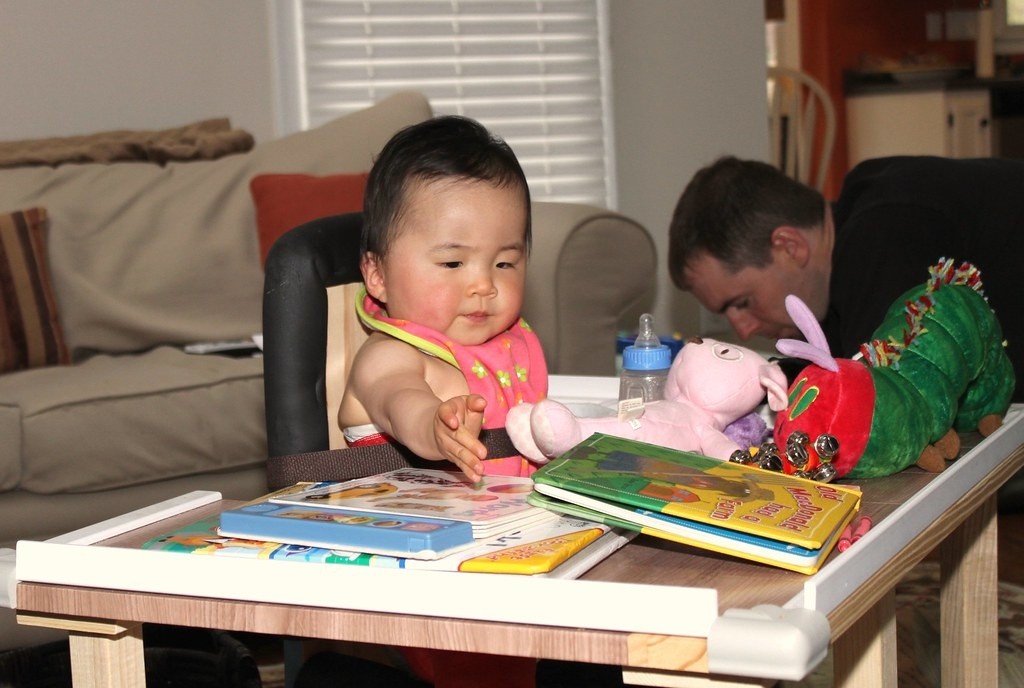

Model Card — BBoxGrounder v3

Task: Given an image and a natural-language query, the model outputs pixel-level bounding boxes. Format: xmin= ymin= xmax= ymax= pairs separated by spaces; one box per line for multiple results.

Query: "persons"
xmin=668 ymin=156 xmax=1023 ymax=511
xmin=336 ymin=115 xmax=548 ymax=483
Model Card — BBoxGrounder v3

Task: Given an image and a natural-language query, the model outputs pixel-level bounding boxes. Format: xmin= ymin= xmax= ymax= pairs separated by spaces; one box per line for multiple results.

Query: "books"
xmin=527 ymin=432 xmax=862 ymax=575
xmin=141 ymin=466 xmax=640 ymax=579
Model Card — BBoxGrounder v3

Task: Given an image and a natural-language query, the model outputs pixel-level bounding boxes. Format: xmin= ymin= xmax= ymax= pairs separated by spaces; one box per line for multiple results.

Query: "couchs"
xmin=0 ymin=91 xmax=658 ymax=653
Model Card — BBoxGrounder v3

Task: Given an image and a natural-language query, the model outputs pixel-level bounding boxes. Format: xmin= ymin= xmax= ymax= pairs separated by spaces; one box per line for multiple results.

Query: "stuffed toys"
xmin=773 ymin=259 xmax=1016 ymax=484
xmin=506 ymin=337 xmax=788 ymax=464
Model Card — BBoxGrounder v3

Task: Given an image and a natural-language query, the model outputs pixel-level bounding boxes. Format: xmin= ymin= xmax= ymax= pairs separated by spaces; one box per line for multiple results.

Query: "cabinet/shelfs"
xmin=846 ymin=92 xmax=992 ymax=172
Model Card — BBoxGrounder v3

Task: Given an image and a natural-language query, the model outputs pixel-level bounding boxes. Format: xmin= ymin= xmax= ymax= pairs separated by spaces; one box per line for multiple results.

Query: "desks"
xmin=12 ymin=397 xmax=1023 ymax=688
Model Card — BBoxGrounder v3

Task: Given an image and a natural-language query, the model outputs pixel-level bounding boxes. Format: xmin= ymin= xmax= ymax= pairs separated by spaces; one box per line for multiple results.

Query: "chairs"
xmin=767 ymin=66 xmax=836 ymax=196
xmin=262 ymin=212 xmax=370 ymax=494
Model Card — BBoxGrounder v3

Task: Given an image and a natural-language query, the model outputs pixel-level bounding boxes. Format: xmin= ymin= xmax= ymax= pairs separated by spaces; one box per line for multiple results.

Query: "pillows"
xmin=248 ymin=170 xmax=369 ymax=267
xmin=0 ymin=207 xmax=69 ymax=375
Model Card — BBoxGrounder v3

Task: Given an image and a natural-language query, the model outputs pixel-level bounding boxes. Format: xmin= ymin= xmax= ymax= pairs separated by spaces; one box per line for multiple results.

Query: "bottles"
xmin=617 ymin=313 xmax=672 ymax=407
